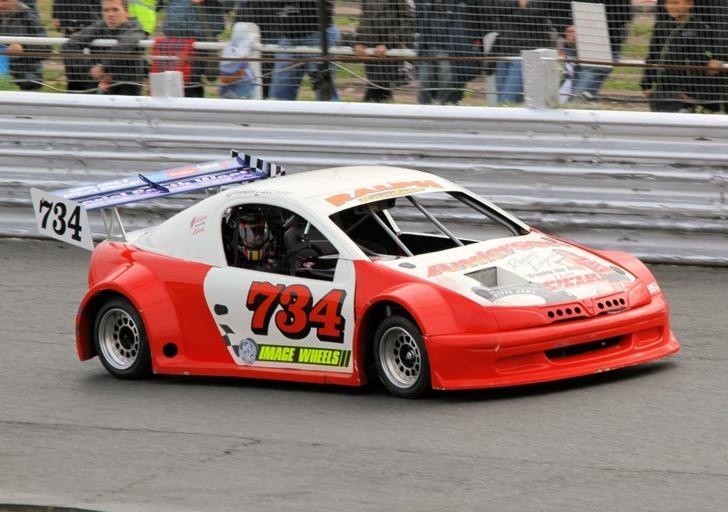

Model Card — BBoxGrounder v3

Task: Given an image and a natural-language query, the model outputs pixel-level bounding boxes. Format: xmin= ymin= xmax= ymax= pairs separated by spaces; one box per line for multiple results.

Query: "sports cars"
xmin=28 ymin=145 xmax=679 ymax=395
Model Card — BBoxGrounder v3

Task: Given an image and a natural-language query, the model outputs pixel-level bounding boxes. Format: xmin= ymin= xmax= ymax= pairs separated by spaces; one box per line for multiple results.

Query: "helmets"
xmin=236 ymin=216 xmax=273 ymax=261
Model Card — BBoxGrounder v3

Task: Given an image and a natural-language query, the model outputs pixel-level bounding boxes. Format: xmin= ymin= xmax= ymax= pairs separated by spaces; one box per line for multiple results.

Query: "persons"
xmin=1 ymin=0 xmax=728 ymax=115
xmin=229 ymin=208 xmax=314 ymax=278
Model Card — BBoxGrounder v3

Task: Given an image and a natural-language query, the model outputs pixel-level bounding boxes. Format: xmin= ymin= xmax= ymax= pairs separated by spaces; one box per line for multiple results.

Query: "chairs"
xmin=284 ymin=213 xmax=377 ymax=282
